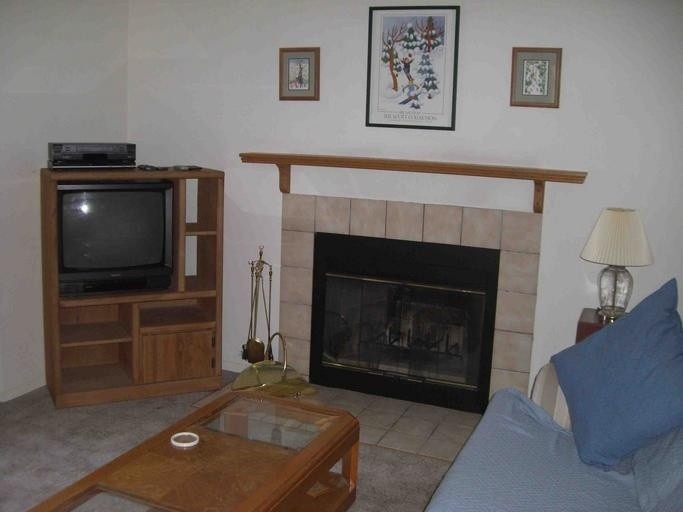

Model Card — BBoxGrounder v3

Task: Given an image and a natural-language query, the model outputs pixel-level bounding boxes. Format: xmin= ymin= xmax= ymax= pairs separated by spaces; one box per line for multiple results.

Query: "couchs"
xmin=421 ymin=362 xmax=683 ymax=511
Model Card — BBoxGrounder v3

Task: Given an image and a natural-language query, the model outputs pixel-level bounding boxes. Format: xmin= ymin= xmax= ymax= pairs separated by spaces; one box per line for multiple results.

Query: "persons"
xmin=399 ymin=57 xmax=416 ymax=84
xmin=402 ymin=79 xmax=419 ymax=97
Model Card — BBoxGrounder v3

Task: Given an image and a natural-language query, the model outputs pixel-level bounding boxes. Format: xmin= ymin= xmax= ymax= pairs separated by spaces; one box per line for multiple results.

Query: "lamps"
xmin=579 ymin=207 xmax=654 ymax=322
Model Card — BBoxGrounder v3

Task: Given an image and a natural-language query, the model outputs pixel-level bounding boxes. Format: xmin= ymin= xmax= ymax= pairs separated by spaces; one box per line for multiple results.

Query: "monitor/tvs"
xmin=56 ymin=179 xmax=175 ymax=295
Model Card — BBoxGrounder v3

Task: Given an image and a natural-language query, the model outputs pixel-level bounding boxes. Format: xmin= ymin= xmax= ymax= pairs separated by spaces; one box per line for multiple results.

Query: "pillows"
xmin=550 ymin=278 xmax=683 ymax=473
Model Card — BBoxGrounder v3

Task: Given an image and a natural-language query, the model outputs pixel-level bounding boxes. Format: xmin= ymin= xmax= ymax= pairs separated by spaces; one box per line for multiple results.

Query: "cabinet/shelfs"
xmin=39 ymin=166 xmax=225 ymax=410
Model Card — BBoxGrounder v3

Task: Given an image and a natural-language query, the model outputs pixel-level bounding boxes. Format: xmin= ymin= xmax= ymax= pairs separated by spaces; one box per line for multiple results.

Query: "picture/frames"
xmin=364 ymin=5 xmax=461 ymax=132
xmin=509 ymin=47 xmax=562 ymax=108
xmin=277 ymin=47 xmax=320 ymax=102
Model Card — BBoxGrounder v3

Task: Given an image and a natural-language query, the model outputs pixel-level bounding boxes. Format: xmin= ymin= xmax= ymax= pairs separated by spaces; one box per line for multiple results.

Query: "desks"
xmin=575 ymin=308 xmax=628 ymax=347
